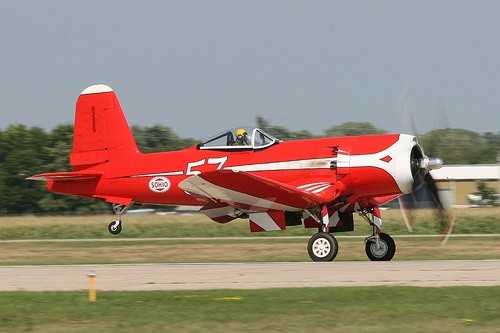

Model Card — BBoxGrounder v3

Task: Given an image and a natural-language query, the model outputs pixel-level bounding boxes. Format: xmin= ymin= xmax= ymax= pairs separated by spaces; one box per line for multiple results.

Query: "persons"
xmin=231 ymin=128 xmax=248 ymax=146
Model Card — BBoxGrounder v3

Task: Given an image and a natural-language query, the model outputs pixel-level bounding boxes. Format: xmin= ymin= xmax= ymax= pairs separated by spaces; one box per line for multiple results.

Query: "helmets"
xmin=236 ymin=129 xmax=246 ymax=139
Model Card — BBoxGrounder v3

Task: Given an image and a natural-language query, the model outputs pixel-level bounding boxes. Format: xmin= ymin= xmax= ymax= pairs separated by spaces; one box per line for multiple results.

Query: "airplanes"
xmin=24 ymin=84 xmax=445 ymax=263
